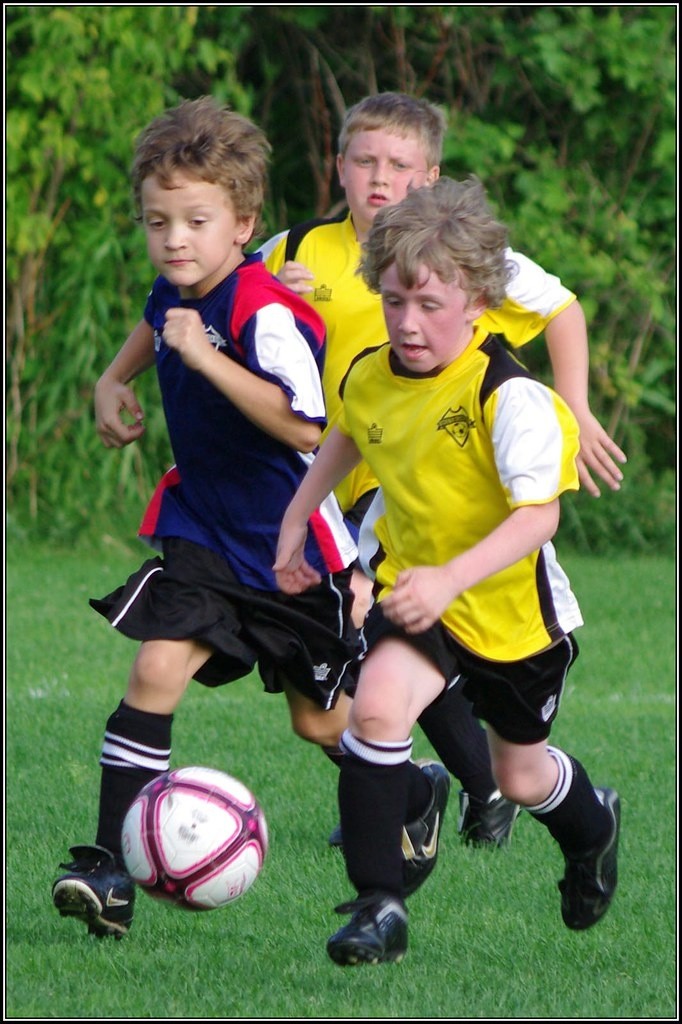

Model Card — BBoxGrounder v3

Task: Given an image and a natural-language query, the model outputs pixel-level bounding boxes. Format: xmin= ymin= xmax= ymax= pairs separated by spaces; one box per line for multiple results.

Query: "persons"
xmin=273 ymin=178 xmax=623 ymax=969
xmin=249 ymin=92 xmax=628 ymax=848
xmin=48 ymin=100 xmax=450 ymax=938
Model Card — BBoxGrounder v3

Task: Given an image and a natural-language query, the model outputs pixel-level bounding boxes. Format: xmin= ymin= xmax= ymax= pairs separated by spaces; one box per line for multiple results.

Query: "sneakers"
xmin=558 ymin=786 xmax=621 ymax=930
xmin=459 ymin=789 xmax=522 ymax=849
xmin=327 ymin=894 xmax=408 ymax=968
xmin=404 ymin=757 xmax=450 ymax=898
xmin=329 ymin=824 xmax=341 ymax=846
xmin=52 ymin=845 xmax=138 ymax=941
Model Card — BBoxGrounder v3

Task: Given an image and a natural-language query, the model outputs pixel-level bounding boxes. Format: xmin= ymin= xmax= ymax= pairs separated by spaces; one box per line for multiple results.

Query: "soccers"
xmin=119 ymin=765 xmax=270 ymax=913
